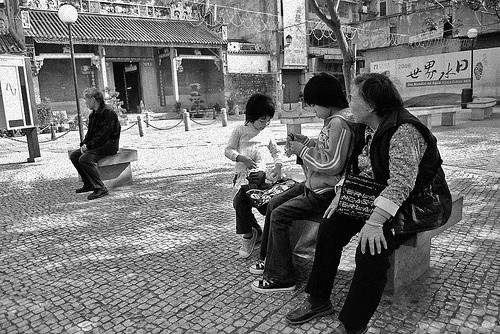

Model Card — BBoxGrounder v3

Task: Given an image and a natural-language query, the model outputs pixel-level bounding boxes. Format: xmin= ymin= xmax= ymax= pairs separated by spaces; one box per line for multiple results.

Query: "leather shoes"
xmin=76 ymin=185 xmax=93 ymax=193
xmin=87 ymin=188 xmax=109 ymax=200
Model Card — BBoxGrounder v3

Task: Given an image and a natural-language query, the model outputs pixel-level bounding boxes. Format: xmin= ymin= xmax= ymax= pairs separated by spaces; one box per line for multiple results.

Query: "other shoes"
xmin=286 ymin=299 xmax=334 ymax=325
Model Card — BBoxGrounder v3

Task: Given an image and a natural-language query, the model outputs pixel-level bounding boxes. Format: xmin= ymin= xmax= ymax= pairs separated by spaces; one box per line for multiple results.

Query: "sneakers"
xmin=250 ymin=261 xmax=265 ymax=274
xmin=239 ymin=227 xmax=261 ymax=258
xmin=252 ymin=277 xmax=296 ymax=293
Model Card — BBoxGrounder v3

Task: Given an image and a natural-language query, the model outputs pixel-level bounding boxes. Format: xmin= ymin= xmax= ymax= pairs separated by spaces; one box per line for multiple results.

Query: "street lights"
xmin=467 ymin=28 xmax=478 ymax=93
xmin=58 ymin=3 xmax=84 ymax=141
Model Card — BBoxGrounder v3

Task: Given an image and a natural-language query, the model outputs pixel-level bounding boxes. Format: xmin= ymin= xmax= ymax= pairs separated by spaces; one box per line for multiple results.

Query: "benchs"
xmin=68 ymin=147 xmax=138 ymax=188
xmin=280 ymin=114 xmax=326 ymax=137
xmin=466 ymin=97 xmax=497 ymax=121
xmin=405 ymin=105 xmax=463 ymax=129
xmin=290 ymin=193 xmax=465 ymax=298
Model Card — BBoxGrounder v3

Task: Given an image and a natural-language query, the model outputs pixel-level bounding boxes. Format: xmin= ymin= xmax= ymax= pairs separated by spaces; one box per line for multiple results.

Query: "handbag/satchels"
xmin=335 ymin=174 xmax=400 ymax=228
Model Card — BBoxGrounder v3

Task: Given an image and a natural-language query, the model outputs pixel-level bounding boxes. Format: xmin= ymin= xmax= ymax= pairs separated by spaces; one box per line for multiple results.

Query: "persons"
xmin=284 ymin=72 xmax=452 ymax=334
xmin=223 ymin=92 xmax=283 ymax=259
xmin=249 ymin=71 xmax=356 ymax=292
xmin=70 ymin=86 xmax=120 ymax=200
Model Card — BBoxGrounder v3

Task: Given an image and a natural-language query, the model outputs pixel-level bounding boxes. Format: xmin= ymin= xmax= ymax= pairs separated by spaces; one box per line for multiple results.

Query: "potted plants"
xmin=55 ymin=112 xmax=70 ymax=132
xmin=37 ymin=104 xmax=53 ymax=134
xmin=189 ymin=83 xmax=205 ymax=118
